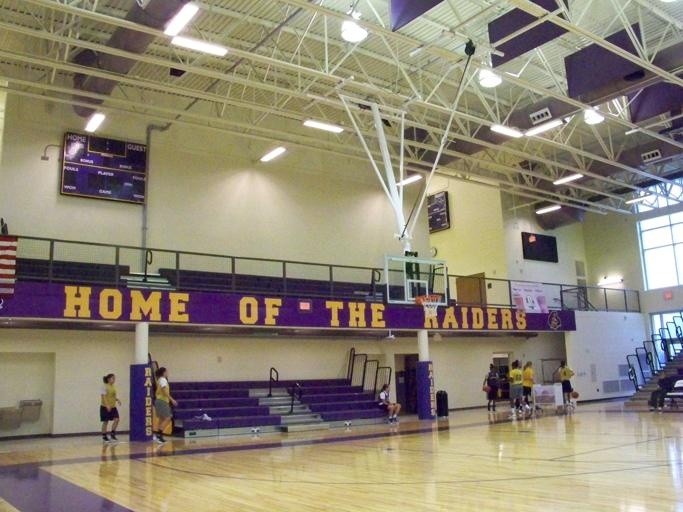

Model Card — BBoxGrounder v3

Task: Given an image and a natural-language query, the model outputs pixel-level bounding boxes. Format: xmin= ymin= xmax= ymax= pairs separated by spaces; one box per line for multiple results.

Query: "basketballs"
xmin=536 ymin=390 xmax=556 ymax=406
xmin=572 ymin=392 xmax=579 ymax=399
xmin=483 ymin=385 xmax=491 ymax=392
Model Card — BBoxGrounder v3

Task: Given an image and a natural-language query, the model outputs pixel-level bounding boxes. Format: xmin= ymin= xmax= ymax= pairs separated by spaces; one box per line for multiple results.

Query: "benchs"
xmin=159 ymin=267 xmax=430 ymax=300
xmin=16 ymin=256 xmax=129 ymax=289
xmin=165 ymin=378 xmax=389 ymax=440
xmin=626 ymin=350 xmax=683 ymax=410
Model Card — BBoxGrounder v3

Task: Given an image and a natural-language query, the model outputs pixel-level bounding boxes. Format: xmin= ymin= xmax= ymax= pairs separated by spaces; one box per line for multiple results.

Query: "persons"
xmin=558 ymin=360 xmax=574 ymax=403
xmin=100 ymin=373 xmax=121 ymax=442
xmin=378 ymin=384 xmax=402 ymax=424
xmin=483 ymin=360 xmax=535 ymax=416
xmin=649 ymin=368 xmax=683 ymax=411
xmin=152 ymin=367 xmax=178 ymax=444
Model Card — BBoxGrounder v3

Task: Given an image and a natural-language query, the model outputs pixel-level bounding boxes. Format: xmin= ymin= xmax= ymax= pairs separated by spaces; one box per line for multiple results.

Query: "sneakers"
xmin=488 ymin=403 xmax=496 ymax=411
xmin=650 ymin=406 xmax=663 ymax=411
xmin=510 ymin=405 xmax=541 ymax=417
xmin=389 ymin=417 xmax=399 ymax=424
xmin=156 ymin=436 xmax=168 ymax=444
xmin=103 ymin=436 xmax=118 ymax=442
